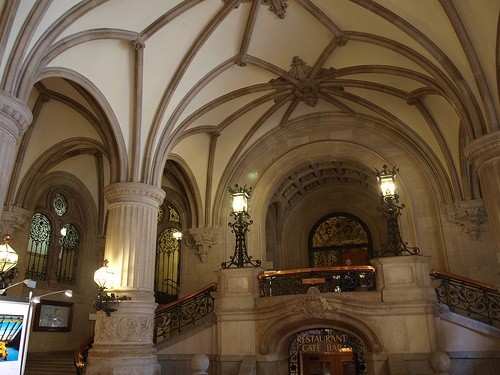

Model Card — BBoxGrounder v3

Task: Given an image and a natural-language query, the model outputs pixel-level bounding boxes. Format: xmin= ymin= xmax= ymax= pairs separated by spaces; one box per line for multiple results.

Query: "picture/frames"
xmin=31 ymin=299 xmax=75 ymax=333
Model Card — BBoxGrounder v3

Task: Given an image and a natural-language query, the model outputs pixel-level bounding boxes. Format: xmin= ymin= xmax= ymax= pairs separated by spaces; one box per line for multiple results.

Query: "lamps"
xmin=91 ymin=258 xmax=131 ymax=318
xmin=173 ymin=231 xmax=183 ymax=241
xmin=372 ymin=164 xmax=422 ymax=258
xmin=0 ymin=278 xmax=37 ymax=295
xmin=0 ymin=234 xmax=20 ymax=297
xmin=31 ymin=289 xmax=73 ymax=304
xmin=218 ymin=182 xmax=262 ymax=268
